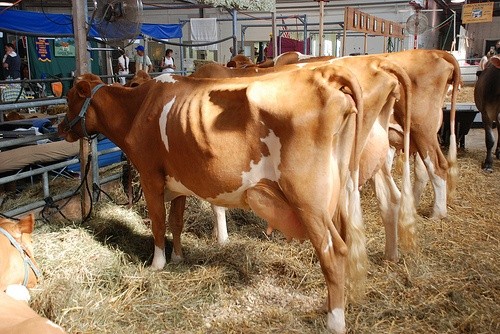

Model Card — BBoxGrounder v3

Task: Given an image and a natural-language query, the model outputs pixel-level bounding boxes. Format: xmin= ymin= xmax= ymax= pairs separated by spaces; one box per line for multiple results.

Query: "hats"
xmin=135 ymin=46 xmax=144 ymax=51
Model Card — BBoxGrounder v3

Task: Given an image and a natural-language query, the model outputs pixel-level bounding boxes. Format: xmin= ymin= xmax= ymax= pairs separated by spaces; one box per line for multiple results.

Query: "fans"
xmin=85 ymin=0 xmax=144 ymax=50
xmin=406 ymin=13 xmax=429 ymax=35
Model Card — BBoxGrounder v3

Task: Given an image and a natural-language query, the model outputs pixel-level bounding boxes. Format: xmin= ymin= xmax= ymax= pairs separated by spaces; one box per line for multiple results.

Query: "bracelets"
xmin=169 ymin=65 xmax=170 ymax=67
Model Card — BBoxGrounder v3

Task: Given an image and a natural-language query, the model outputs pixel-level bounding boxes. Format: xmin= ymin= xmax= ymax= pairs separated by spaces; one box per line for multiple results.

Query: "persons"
xmin=117 ymin=49 xmax=129 ymax=86
xmin=134 ymin=46 xmax=152 ymax=73
xmin=0 ymin=43 xmax=20 ymax=79
xmin=160 ymin=49 xmax=175 ymax=72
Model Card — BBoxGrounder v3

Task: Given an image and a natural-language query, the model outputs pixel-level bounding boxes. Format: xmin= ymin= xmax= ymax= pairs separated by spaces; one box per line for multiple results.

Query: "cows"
xmin=0 ymin=214 xmax=66 ymax=333
xmin=56 ymin=48 xmax=461 ymax=333
xmin=473 ymin=54 xmax=500 ymax=169
